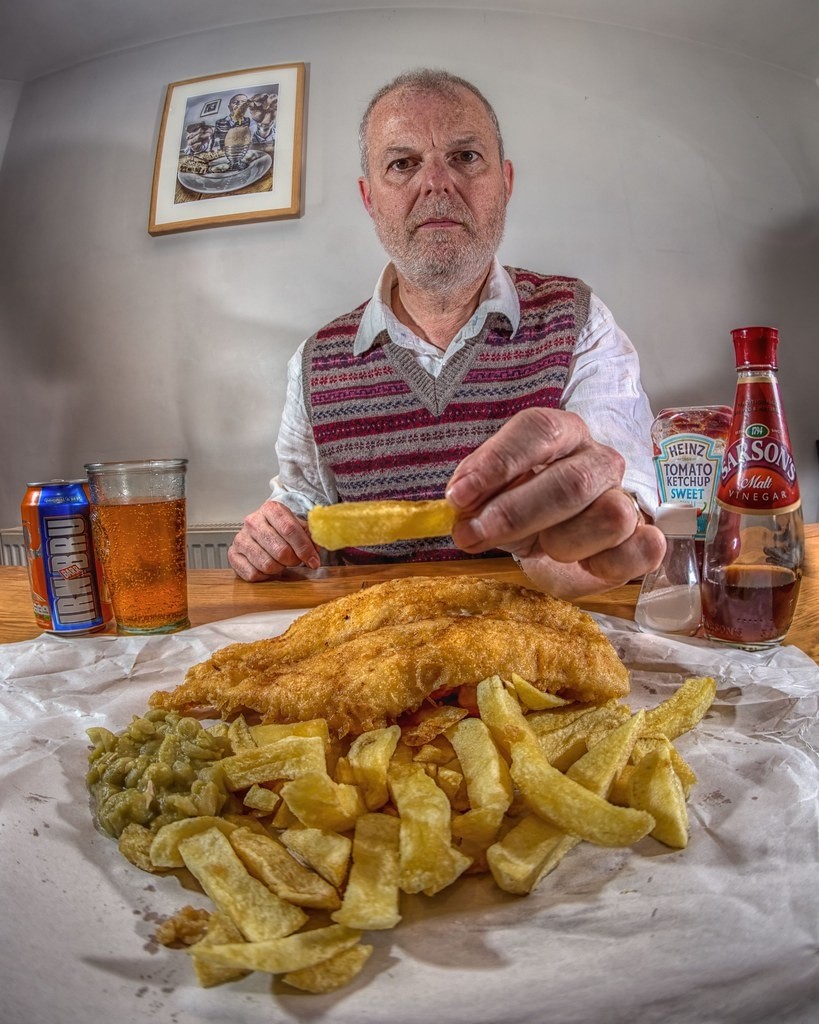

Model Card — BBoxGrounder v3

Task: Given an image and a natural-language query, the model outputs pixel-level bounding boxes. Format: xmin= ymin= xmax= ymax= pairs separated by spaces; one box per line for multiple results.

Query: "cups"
xmin=84 ymin=458 xmax=189 ymax=633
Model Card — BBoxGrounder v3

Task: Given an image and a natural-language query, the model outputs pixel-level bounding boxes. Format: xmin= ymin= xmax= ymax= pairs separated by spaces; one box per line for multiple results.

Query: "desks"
xmin=1 ymin=520 xmax=819 ymax=660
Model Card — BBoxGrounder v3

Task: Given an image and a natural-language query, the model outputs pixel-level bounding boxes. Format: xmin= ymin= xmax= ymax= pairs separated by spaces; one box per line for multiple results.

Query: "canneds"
xmin=20 ymin=478 xmax=107 ymax=637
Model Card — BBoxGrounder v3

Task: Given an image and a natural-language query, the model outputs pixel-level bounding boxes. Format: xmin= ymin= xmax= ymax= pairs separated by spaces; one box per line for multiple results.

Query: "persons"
xmin=228 ymin=69 xmax=666 ymax=600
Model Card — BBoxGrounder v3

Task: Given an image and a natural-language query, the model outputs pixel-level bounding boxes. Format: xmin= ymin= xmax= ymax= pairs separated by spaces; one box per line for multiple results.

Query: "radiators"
xmin=1 ymin=524 xmax=243 ymax=570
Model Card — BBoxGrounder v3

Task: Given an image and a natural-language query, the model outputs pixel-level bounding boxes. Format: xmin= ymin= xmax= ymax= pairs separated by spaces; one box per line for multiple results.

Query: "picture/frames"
xmin=149 ymin=62 xmax=305 ymax=236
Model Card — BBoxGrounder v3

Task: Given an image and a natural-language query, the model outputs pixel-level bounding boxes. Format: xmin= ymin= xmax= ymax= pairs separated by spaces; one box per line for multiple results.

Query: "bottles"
xmin=635 ymin=499 xmax=703 ymax=639
xmin=651 ymin=405 xmax=741 ymax=581
xmin=702 ymin=326 xmax=806 ymax=648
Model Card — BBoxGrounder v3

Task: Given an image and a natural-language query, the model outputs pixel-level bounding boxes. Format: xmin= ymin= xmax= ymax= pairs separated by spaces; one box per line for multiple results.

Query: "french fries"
xmin=148 ymin=673 xmax=716 ymax=992
xmin=308 ymin=501 xmax=484 ymax=551
